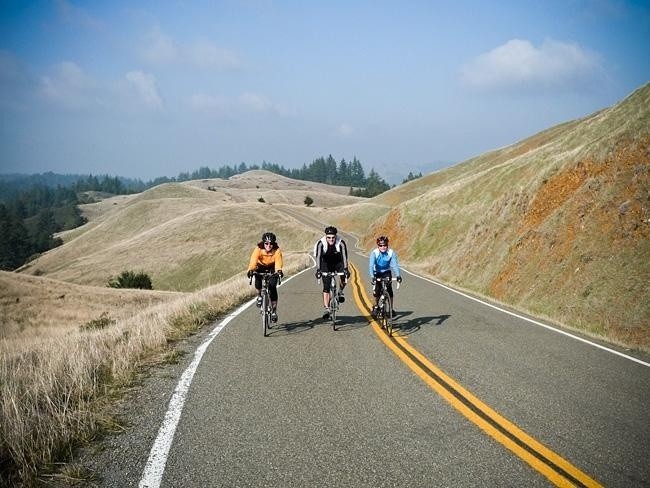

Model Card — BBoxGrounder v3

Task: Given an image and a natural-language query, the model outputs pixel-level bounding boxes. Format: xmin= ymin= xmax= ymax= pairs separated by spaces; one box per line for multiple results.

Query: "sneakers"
xmin=339 ymin=293 xmax=344 ymax=303
xmin=272 ymin=312 xmax=278 ymax=321
xmin=390 ymin=310 xmax=396 ymax=317
xmin=323 ymin=312 xmax=329 ymax=319
xmin=373 ymin=305 xmax=379 ymax=316
xmin=257 ymin=296 xmax=262 ymax=307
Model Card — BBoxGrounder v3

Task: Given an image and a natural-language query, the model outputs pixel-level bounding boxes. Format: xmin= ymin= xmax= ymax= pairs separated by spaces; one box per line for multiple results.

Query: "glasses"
xmin=379 ymin=245 xmax=387 ymax=247
xmin=265 ymin=243 xmax=274 ymax=245
xmin=327 ymin=237 xmax=335 ymax=239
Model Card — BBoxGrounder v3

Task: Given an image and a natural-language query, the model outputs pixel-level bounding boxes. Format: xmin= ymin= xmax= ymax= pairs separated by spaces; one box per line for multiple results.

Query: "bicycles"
xmin=317 ymin=272 xmax=347 ymax=331
xmin=249 ymin=271 xmax=281 ymax=336
xmin=372 ymin=277 xmax=400 ymax=337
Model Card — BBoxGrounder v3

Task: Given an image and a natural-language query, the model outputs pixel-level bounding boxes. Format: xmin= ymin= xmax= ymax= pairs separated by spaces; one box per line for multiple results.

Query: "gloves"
xmin=397 ymin=276 xmax=402 ymax=283
xmin=247 ymin=270 xmax=253 ymax=278
xmin=370 ymin=278 xmax=374 ymax=284
xmin=344 ymin=270 xmax=349 ymax=278
xmin=278 ymin=272 xmax=283 ymax=277
xmin=315 ymin=272 xmax=322 ymax=279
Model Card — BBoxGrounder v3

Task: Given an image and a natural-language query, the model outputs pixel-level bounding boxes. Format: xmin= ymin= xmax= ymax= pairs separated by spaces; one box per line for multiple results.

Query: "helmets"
xmin=325 ymin=226 xmax=336 ymax=234
xmin=377 ymin=236 xmax=388 ymax=244
xmin=262 ymin=232 xmax=277 ymax=242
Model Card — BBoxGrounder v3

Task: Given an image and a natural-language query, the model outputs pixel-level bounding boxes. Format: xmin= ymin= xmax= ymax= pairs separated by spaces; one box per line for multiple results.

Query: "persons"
xmin=313 ymin=226 xmax=348 ymax=320
xmin=368 ymin=235 xmax=400 ymax=316
xmin=247 ymin=233 xmax=283 ymax=323
